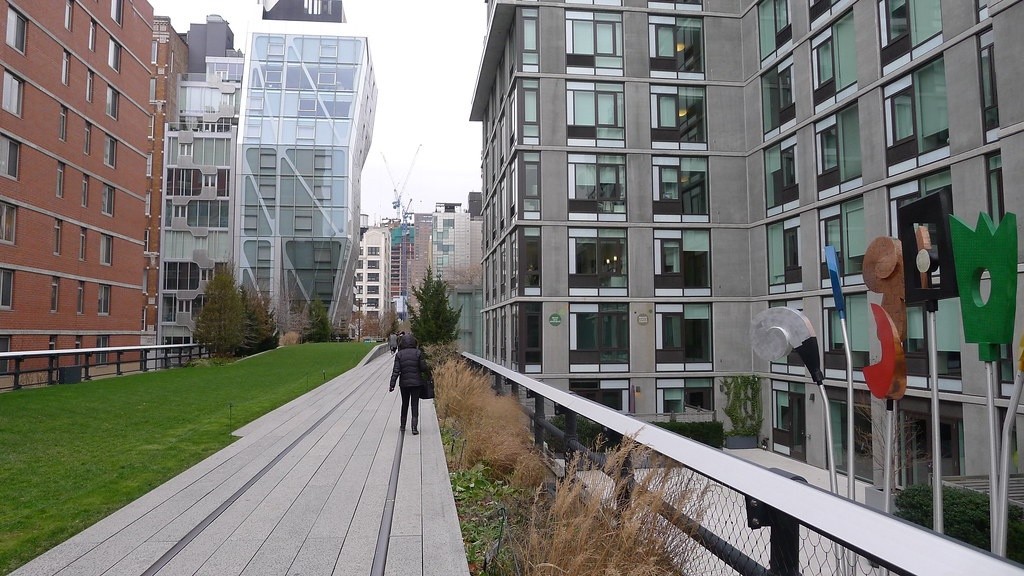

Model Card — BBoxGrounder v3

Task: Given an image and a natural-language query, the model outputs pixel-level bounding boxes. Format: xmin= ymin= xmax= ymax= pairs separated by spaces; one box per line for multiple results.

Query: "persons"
xmin=388 ymin=336 xmax=432 ymax=435
xmin=388 ymin=331 xmax=405 ymax=355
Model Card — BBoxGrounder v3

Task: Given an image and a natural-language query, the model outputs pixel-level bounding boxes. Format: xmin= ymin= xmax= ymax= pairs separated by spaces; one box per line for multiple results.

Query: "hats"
xmin=400 ymin=331 xmax=405 ymax=336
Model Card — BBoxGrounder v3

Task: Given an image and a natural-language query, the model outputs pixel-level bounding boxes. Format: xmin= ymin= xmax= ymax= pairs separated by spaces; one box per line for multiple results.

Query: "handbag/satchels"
xmin=420 ymin=369 xmax=435 ymax=399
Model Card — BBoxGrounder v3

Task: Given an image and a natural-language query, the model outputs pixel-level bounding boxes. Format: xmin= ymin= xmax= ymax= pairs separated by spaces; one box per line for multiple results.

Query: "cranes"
xmin=380 ymin=143 xmax=422 ymax=223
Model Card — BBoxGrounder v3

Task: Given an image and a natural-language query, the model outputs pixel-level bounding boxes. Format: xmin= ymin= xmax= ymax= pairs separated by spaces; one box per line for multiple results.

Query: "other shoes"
xmin=401 ymin=425 xmax=405 ymax=431
xmin=412 ymin=426 xmax=419 ymax=434
